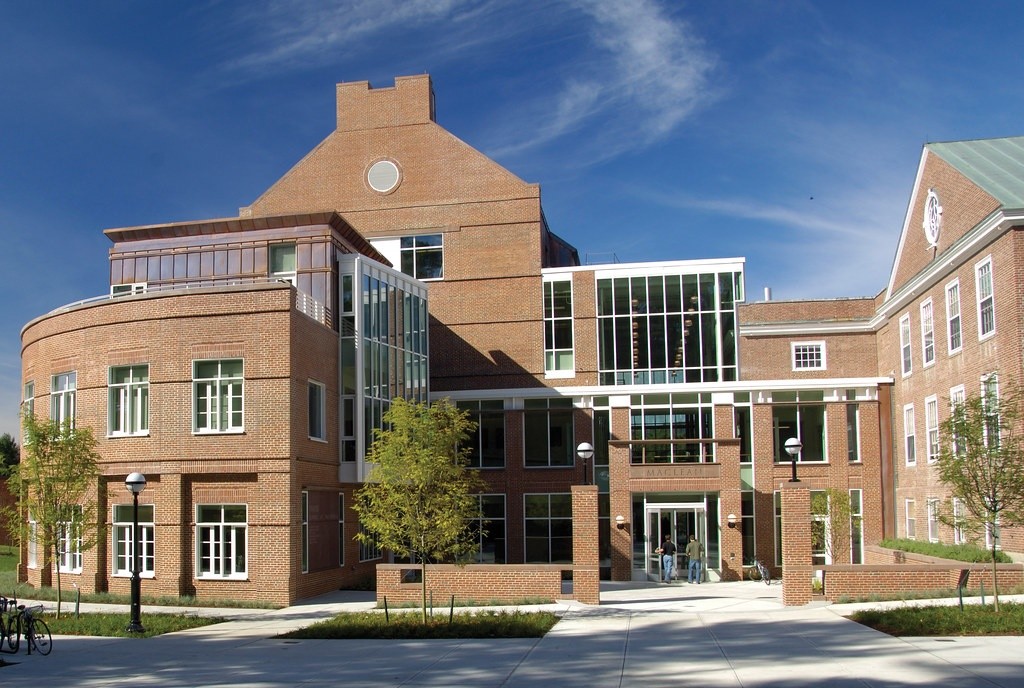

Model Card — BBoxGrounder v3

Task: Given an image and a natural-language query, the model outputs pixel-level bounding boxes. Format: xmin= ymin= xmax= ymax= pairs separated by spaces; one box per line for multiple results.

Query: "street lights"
xmin=124 ymin=472 xmax=147 ymax=633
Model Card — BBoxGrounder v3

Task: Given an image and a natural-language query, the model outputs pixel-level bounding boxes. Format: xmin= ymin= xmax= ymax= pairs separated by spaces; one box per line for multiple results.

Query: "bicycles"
xmin=749 ymin=554 xmax=770 ymax=585
xmin=0 ymin=596 xmax=52 ymax=655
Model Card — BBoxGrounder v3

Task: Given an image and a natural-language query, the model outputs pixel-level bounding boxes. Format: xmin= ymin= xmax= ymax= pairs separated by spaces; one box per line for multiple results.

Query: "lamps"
xmin=632 ymin=299 xmax=639 ymax=378
xmin=672 ymin=297 xmax=699 ymax=376
xmin=728 ymin=514 xmax=736 ymax=528
xmin=615 ymin=516 xmax=624 ymax=529
xmin=784 ymin=438 xmax=803 ymax=482
xmin=576 ymin=442 xmax=594 ymax=484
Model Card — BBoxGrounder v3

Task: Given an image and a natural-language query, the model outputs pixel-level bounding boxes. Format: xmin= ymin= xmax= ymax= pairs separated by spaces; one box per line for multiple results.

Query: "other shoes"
xmin=696 ymin=581 xmax=700 ymax=584
xmin=665 ymin=580 xmax=671 ymax=584
xmin=688 ymin=581 xmax=693 ymax=583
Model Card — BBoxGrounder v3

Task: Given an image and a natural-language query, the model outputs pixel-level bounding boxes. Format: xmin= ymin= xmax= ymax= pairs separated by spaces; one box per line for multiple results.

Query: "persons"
xmin=655 ymin=535 xmax=676 ymax=584
xmin=686 ymin=535 xmax=705 ymax=584
